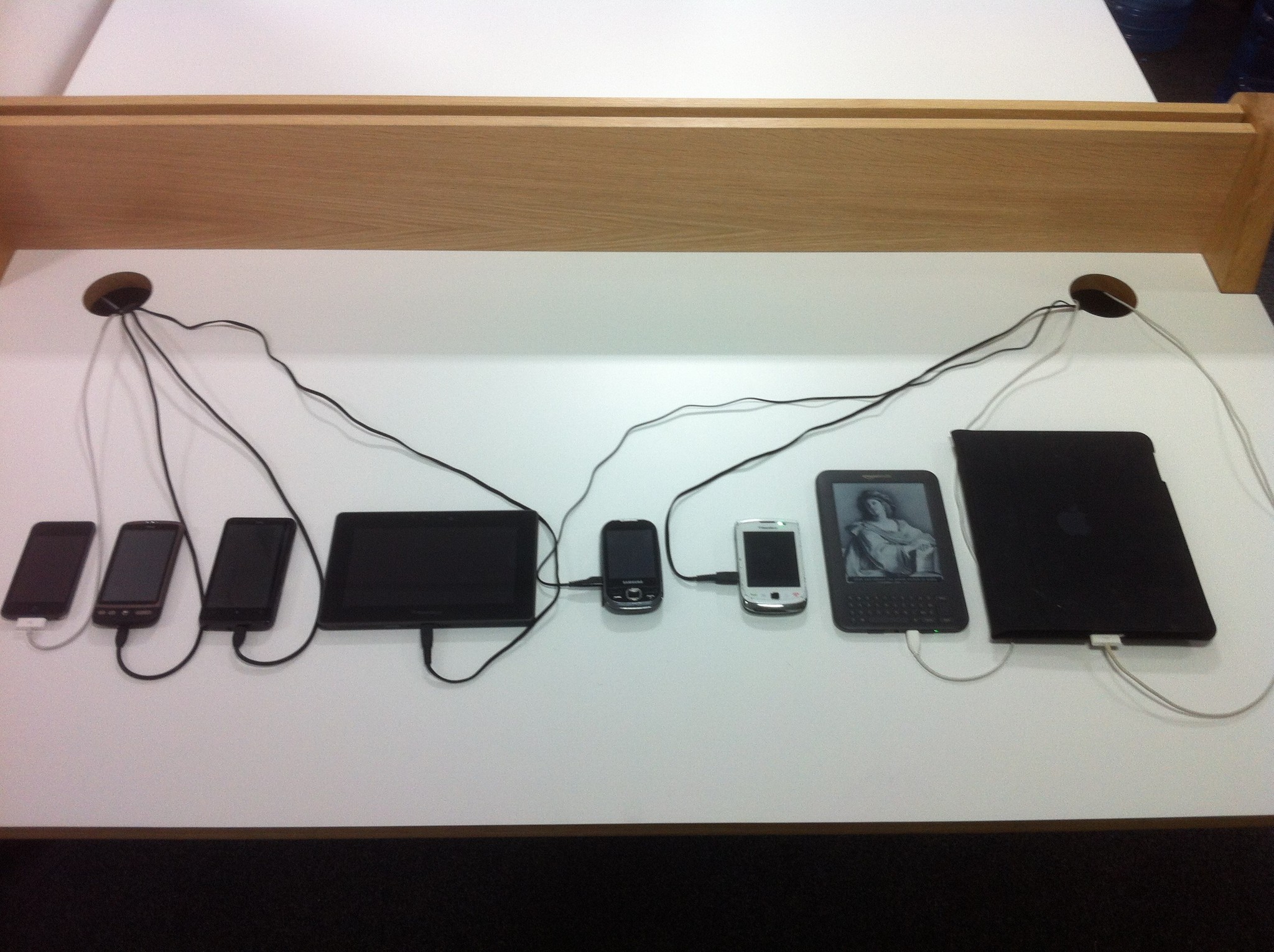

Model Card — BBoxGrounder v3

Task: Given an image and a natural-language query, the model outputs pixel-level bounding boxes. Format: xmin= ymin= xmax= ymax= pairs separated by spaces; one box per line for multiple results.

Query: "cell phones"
xmin=734 ymin=518 xmax=809 ymax=616
xmin=198 ymin=517 xmax=297 ymax=631
xmin=599 ymin=519 xmax=664 ymax=615
xmin=815 ymin=471 xmax=969 ymax=635
xmin=1 ymin=521 xmax=95 ymax=621
xmin=92 ymin=521 xmax=185 ymax=628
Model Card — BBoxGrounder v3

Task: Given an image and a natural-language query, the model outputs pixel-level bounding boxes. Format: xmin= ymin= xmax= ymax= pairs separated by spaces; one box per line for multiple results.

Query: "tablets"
xmin=950 ymin=429 xmax=1217 ymax=643
xmin=315 ymin=510 xmax=538 ymax=630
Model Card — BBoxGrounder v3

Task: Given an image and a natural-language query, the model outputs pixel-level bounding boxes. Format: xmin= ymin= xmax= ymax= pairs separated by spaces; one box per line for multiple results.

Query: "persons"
xmin=841 ymin=489 xmax=940 ymax=575
